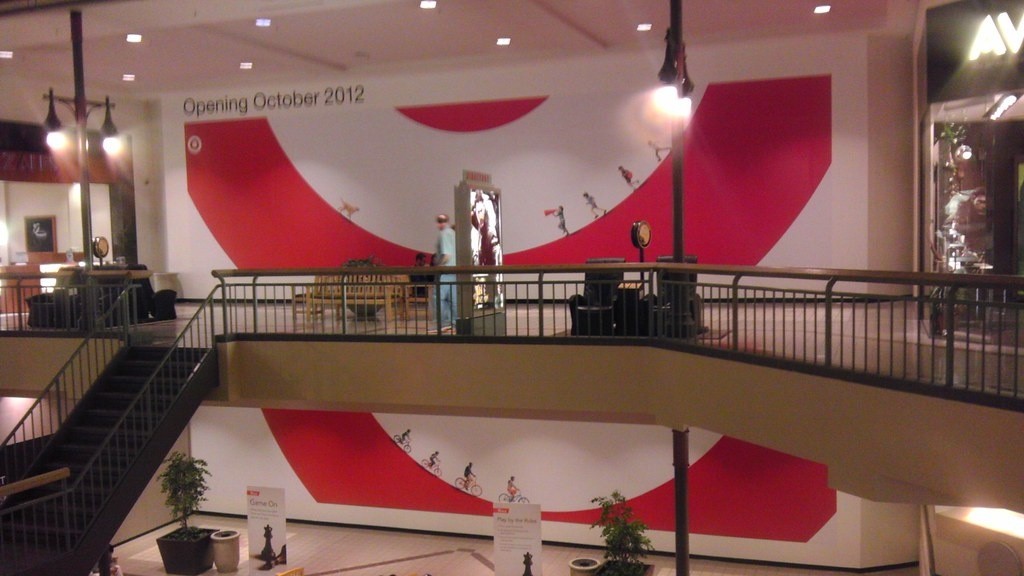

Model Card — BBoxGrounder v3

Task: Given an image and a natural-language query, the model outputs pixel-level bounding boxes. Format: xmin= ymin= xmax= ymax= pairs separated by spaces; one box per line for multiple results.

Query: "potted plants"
xmin=584 ymin=492 xmax=664 ymax=576
xmin=150 ymin=443 xmax=235 ymax=575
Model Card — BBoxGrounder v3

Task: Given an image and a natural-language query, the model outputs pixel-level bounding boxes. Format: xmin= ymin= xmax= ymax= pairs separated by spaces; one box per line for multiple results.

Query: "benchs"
xmin=291 ymin=267 xmax=438 ymax=323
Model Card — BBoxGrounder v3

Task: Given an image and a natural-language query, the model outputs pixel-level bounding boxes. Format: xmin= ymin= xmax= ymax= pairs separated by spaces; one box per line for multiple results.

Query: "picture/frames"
xmin=24 ymin=216 xmax=57 ymax=254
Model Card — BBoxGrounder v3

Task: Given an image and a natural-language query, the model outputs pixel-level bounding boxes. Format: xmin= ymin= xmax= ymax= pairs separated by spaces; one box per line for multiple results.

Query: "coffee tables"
xmin=617 ymin=282 xmax=645 ymax=335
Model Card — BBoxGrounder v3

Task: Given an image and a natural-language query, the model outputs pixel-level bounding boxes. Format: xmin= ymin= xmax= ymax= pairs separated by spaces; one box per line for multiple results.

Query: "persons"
xmin=428 ymin=451 xmax=440 ymax=467
xmin=583 ymin=192 xmax=607 ymax=218
xmin=507 ymin=476 xmax=520 ymax=496
xmin=410 ymin=214 xmax=458 ymax=332
xmin=647 ymin=139 xmax=671 ymax=162
xmin=618 ymin=166 xmax=640 ymax=187
xmin=464 ymin=462 xmax=476 ymax=491
xmin=552 ymin=205 xmax=570 ymax=236
xmin=942 ymin=188 xmax=987 ymax=249
xmin=401 ymin=429 xmax=411 ymax=442
xmin=471 ymin=189 xmax=500 ymax=265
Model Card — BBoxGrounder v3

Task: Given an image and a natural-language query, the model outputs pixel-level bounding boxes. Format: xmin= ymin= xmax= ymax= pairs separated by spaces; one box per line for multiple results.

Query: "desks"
xmin=151 ymin=272 xmax=179 ymax=293
xmin=58 ymin=262 xmax=154 ymax=329
xmin=0 ymin=264 xmax=44 ymax=314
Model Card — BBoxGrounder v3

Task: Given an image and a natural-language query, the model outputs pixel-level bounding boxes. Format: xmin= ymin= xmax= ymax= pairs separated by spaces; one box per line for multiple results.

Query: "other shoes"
xmin=427 ymin=322 xmax=452 ymax=332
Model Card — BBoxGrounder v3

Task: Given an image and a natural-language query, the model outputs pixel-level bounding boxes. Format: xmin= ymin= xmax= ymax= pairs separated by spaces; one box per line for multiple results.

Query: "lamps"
xmin=934 ymin=131 xmax=973 ymax=160
xmin=45 ymin=86 xmax=126 ymax=154
xmin=653 ymin=23 xmax=695 ymax=121
xmin=11 ymin=251 xmax=30 ymax=265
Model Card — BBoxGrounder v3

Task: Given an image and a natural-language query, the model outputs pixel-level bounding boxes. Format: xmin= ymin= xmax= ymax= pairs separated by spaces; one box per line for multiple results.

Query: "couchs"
xmin=639 ymin=254 xmax=702 ymax=340
xmin=25 ymin=289 xmax=177 ymax=329
xmin=567 ymin=257 xmax=626 ymax=334
